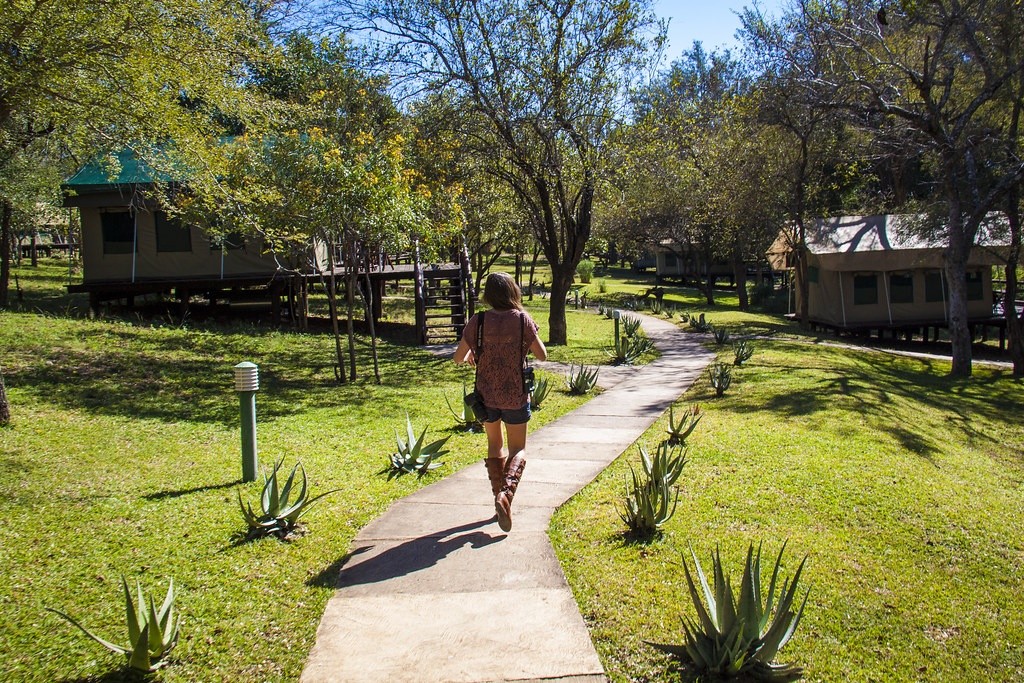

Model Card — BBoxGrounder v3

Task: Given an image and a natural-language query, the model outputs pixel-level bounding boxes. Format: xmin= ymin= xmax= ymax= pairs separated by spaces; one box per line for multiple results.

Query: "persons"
xmin=452 ymin=272 xmax=548 ymax=533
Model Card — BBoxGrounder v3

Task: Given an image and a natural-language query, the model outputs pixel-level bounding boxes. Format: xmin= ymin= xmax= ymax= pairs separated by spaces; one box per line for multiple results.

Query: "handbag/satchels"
xmin=464 ymin=393 xmax=488 ymax=422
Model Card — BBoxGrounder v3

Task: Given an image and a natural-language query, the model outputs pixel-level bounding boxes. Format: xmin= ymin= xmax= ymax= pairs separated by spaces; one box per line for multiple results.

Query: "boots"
xmin=484 ymin=455 xmax=507 ymax=520
xmin=495 ymin=455 xmax=526 ymax=532
